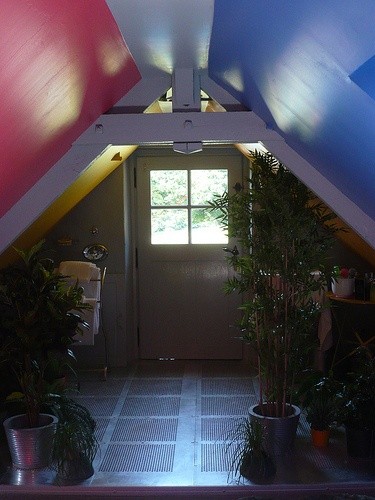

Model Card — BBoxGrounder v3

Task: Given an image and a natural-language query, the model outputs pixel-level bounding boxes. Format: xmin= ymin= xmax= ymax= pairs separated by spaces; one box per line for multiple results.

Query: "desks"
xmin=324 ymin=289 xmax=375 ymax=376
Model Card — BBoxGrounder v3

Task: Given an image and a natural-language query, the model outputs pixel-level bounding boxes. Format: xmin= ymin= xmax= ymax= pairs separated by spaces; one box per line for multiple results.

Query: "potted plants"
xmin=201 ymin=148 xmax=375 ymax=484
xmin=0 ymin=237 xmax=98 ymax=471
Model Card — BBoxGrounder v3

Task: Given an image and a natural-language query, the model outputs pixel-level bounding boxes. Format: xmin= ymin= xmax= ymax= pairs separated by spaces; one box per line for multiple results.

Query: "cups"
xmin=356 ymin=279 xmax=370 ymax=299
xmin=331 ymin=277 xmax=355 ymax=298
